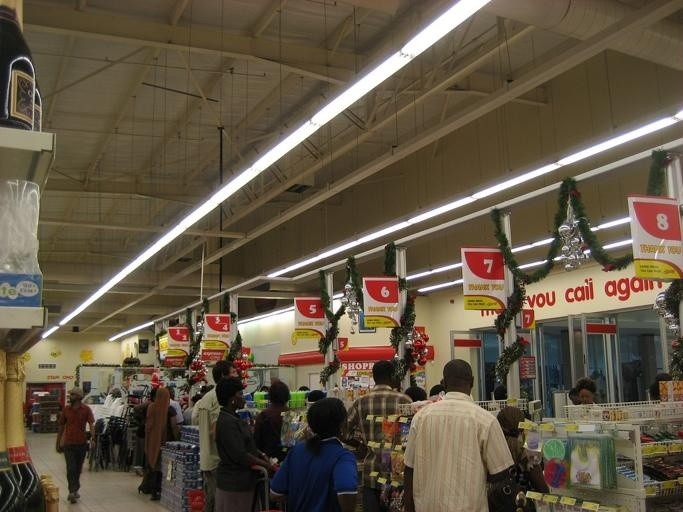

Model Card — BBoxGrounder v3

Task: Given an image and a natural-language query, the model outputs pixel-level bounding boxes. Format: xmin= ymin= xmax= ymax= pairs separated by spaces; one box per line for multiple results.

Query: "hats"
xmin=68 ymin=387 xmax=83 ymax=397
xmin=497 ymin=407 xmax=524 ymax=434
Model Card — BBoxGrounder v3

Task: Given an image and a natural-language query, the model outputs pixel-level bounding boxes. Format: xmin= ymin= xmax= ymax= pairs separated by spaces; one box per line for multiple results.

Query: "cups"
xmin=252 ymin=392 xmax=305 ymax=409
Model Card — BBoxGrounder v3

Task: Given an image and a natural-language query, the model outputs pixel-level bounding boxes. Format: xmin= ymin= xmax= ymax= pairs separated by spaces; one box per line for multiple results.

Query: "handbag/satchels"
xmin=488 ymin=468 xmax=536 ymax=512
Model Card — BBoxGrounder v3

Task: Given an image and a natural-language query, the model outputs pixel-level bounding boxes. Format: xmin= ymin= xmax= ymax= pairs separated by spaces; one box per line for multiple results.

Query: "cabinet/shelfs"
xmin=232 ymin=388 xmax=683 ymax=512
xmin=0 ymin=125 xmax=55 ymax=354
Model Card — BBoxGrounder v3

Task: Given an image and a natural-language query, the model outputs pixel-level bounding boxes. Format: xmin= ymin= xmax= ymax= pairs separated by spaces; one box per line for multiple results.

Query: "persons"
xmin=575 ymin=377 xmax=598 ymax=405
xmin=569 ymin=388 xmax=581 ymax=405
xmin=136 ymin=361 xmax=550 ymax=511
xmin=55 ymin=387 xmax=96 ymax=504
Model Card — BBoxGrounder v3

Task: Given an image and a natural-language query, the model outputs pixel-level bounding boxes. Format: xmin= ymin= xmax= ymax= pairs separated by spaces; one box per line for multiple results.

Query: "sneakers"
xmin=68 ymin=490 xmax=80 ymax=503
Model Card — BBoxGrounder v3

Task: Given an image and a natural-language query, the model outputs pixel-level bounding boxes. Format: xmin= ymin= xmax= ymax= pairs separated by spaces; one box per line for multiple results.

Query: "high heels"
xmin=138 ymin=485 xmax=151 ymax=494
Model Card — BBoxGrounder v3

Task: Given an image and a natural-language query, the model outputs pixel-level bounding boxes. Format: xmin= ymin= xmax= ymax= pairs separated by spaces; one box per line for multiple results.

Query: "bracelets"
xmin=90 ymin=437 xmax=95 ymax=440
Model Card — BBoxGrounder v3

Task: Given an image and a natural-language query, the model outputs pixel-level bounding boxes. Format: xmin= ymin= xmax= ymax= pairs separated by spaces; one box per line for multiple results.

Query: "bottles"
xmin=0 ymin=350 xmax=24 ymax=512
xmin=40 ymin=473 xmax=60 ymax=511
xmin=6 ymin=351 xmax=45 ymax=510
